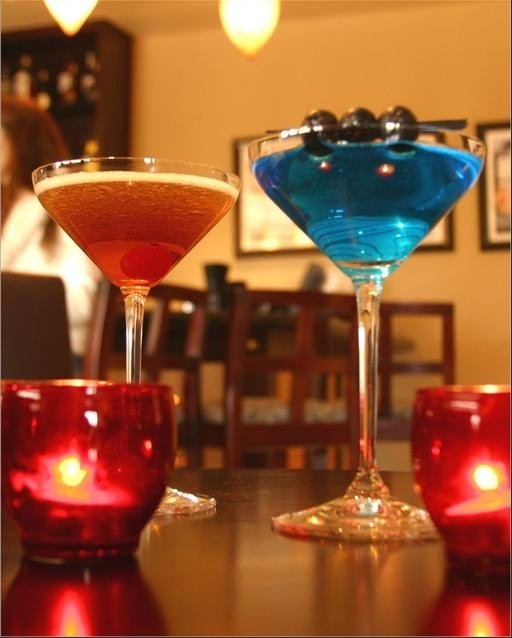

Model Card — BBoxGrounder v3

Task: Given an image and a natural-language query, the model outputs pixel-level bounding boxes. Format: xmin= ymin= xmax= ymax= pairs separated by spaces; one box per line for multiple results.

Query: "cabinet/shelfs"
xmin=3 ymin=16 xmax=134 ymax=173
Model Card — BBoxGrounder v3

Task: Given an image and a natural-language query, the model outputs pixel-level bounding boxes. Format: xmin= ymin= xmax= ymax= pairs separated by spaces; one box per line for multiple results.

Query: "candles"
xmin=437 ymin=465 xmax=509 ymax=547
xmin=11 ymin=455 xmax=140 ymax=553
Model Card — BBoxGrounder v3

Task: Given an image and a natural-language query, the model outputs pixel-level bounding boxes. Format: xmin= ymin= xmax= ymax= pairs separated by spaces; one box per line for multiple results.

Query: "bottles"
xmin=0 ymin=45 xmax=99 ymax=111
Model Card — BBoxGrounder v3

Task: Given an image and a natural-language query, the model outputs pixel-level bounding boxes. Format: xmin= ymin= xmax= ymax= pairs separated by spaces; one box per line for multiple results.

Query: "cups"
xmin=418 ymin=583 xmax=511 ymax=638
xmin=1 ymin=562 xmax=174 ymax=638
xmin=407 ymin=388 xmax=512 ymax=583
xmin=3 ymin=379 xmax=177 ymax=563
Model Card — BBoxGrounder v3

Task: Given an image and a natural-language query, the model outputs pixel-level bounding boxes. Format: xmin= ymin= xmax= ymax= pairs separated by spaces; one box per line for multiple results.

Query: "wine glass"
xmin=244 ymin=125 xmax=488 ymax=538
xmin=29 ymin=156 xmax=238 ymax=519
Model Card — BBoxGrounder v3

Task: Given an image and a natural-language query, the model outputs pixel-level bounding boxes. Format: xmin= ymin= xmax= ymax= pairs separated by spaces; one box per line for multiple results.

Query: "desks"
xmin=0 ymin=462 xmax=512 ymax=637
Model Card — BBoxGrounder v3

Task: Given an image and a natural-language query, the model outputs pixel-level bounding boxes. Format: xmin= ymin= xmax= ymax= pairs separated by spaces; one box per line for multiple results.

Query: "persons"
xmin=1 ymin=91 xmax=102 ymax=380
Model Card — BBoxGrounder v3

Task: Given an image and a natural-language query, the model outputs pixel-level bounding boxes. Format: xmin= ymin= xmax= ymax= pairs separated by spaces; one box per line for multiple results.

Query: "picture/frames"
xmin=230 ymin=128 xmax=456 ymax=262
xmin=473 ymin=119 xmax=512 ymax=254
